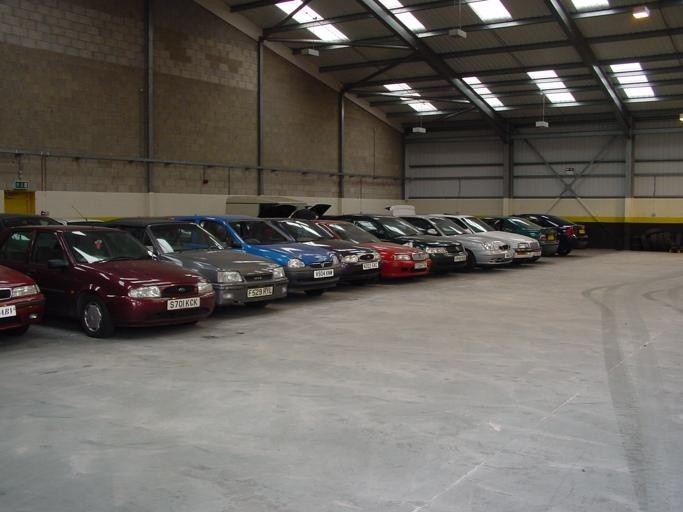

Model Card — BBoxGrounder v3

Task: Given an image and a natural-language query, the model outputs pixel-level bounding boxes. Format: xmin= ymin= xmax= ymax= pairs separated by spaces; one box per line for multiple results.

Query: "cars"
xmin=312 ymin=215 xmax=588 ymax=283
